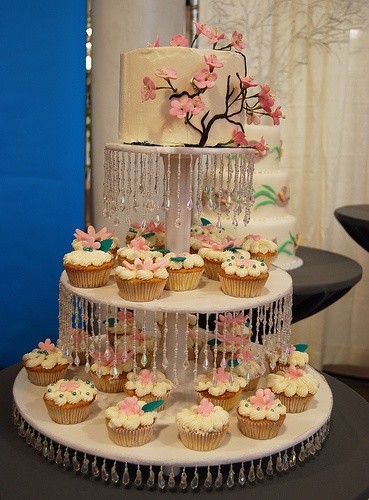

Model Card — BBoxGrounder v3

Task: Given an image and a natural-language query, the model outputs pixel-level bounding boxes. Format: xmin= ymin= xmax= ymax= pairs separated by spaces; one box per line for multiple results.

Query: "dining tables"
xmin=0 ymin=366 xmax=368 ymax=500
xmin=198 ymin=246 xmax=363 ymax=339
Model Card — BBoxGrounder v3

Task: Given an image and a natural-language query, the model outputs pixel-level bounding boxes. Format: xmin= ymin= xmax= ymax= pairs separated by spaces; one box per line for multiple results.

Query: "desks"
xmin=335 ymin=202 xmax=369 ymax=249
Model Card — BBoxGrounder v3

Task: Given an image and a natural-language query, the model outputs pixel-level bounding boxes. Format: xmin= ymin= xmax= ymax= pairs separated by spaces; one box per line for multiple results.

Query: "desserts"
xmin=22 ymin=310 xmax=321 ymax=452
xmin=62 ymin=220 xmax=279 ymax=302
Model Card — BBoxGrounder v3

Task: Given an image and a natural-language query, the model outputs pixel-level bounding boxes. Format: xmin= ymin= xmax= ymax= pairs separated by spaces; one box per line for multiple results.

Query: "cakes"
xmin=192 ymin=125 xmax=300 ymax=266
xmin=118 ymin=22 xmax=281 ymax=146
